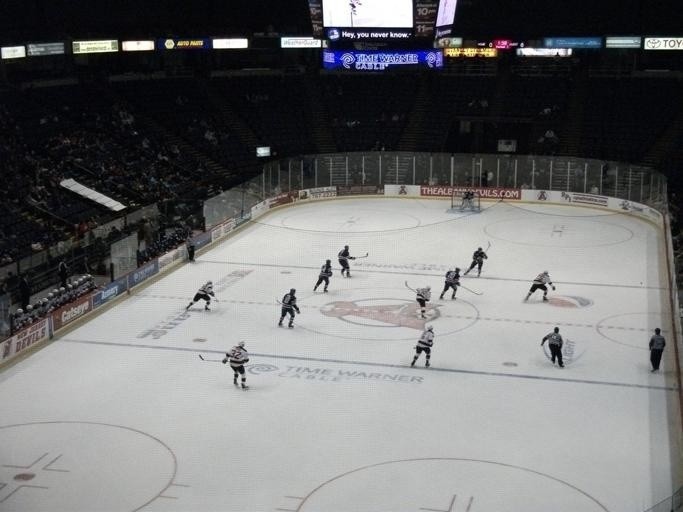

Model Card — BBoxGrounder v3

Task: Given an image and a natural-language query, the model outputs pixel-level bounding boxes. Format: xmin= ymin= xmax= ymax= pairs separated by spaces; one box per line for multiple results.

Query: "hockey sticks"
xmin=406 ymin=281 xmax=417 ymax=293
xmin=485 ymin=242 xmax=491 ymax=253
xmin=355 ymin=253 xmax=369 ymax=259
xmin=199 ymin=354 xmax=224 ymax=362
xmin=276 ymin=298 xmax=282 ymax=304
xmin=459 ymin=284 xmax=483 ymax=296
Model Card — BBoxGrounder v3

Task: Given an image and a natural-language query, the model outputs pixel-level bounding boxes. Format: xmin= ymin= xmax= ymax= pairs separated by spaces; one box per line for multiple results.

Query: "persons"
xmin=541 ymin=327 xmax=565 ymax=367
xmin=410 ymin=324 xmax=434 ymax=367
xmin=222 ymin=340 xmax=249 ymax=389
xmin=650 ymin=328 xmax=666 ymax=372
